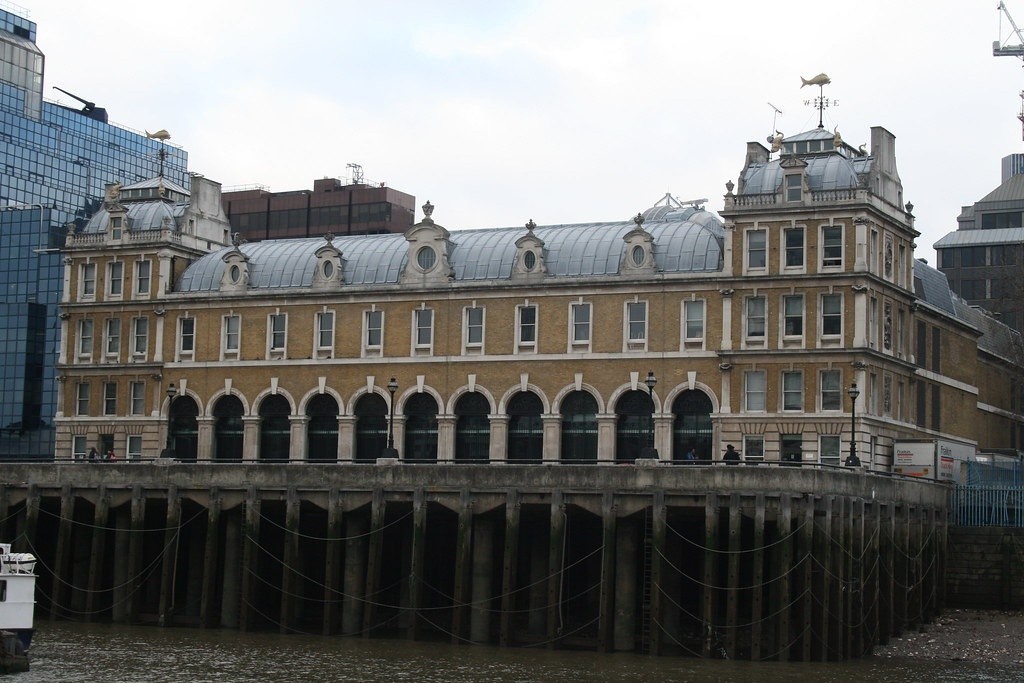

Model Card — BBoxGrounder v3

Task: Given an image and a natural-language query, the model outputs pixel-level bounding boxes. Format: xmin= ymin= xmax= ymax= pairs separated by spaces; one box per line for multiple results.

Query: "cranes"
xmin=989 ymin=1 xmax=1024 ymax=142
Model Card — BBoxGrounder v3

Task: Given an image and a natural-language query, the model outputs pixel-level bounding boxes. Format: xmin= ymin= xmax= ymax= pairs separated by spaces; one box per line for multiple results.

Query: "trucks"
xmin=891 ymin=435 xmax=1024 ymax=486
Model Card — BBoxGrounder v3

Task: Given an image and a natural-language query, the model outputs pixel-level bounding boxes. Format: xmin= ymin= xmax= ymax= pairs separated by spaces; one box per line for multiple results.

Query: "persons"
xmin=687 ymin=447 xmax=699 ymax=465
xmin=88 ymin=447 xmax=116 ymax=463
xmin=723 ymin=445 xmax=742 ymax=464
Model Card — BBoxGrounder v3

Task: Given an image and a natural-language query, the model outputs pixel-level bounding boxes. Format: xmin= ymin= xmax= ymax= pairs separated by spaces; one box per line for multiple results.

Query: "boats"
xmin=0 ymin=541 xmax=40 ymax=677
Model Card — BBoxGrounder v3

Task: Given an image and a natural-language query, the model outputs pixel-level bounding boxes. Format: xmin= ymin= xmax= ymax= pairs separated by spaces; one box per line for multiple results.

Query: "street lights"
xmin=639 ymin=367 xmax=660 ymax=459
xmin=845 ymin=382 xmax=862 ymax=467
xmin=159 ymin=383 xmax=178 ymax=458
xmin=381 ymin=377 xmax=399 ymax=458
